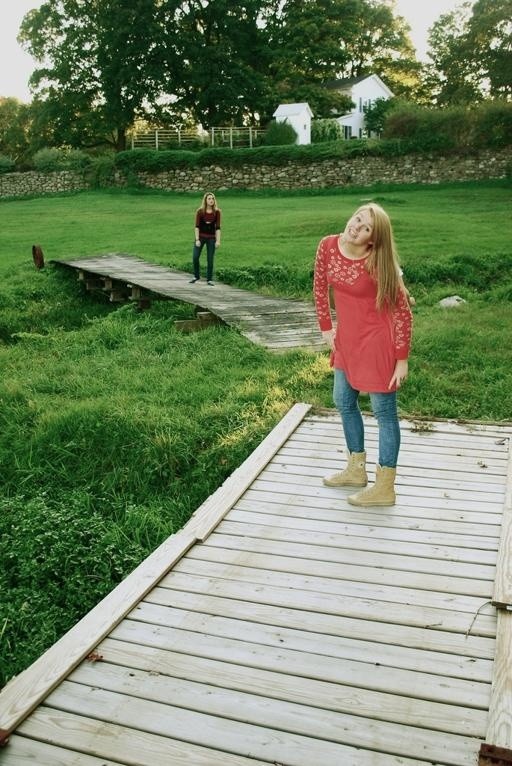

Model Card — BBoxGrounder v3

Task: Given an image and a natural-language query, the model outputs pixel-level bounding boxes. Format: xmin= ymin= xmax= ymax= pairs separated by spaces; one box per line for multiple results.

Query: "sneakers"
xmin=207 ymin=281 xmax=215 ymax=286
xmin=189 ymin=278 xmax=200 ymax=283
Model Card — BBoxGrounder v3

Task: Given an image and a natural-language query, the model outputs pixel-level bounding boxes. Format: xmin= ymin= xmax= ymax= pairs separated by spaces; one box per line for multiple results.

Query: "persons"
xmin=189 ymin=192 xmax=221 ymax=286
xmin=312 ymin=204 xmax=414 ymax=505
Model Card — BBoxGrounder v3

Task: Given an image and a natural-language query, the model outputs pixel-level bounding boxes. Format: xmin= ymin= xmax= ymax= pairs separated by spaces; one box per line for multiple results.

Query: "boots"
xmin=323 ymin=448 xmax=367 ymax=487
xmin=347 ymin=463 xmax=397 ymax=507
